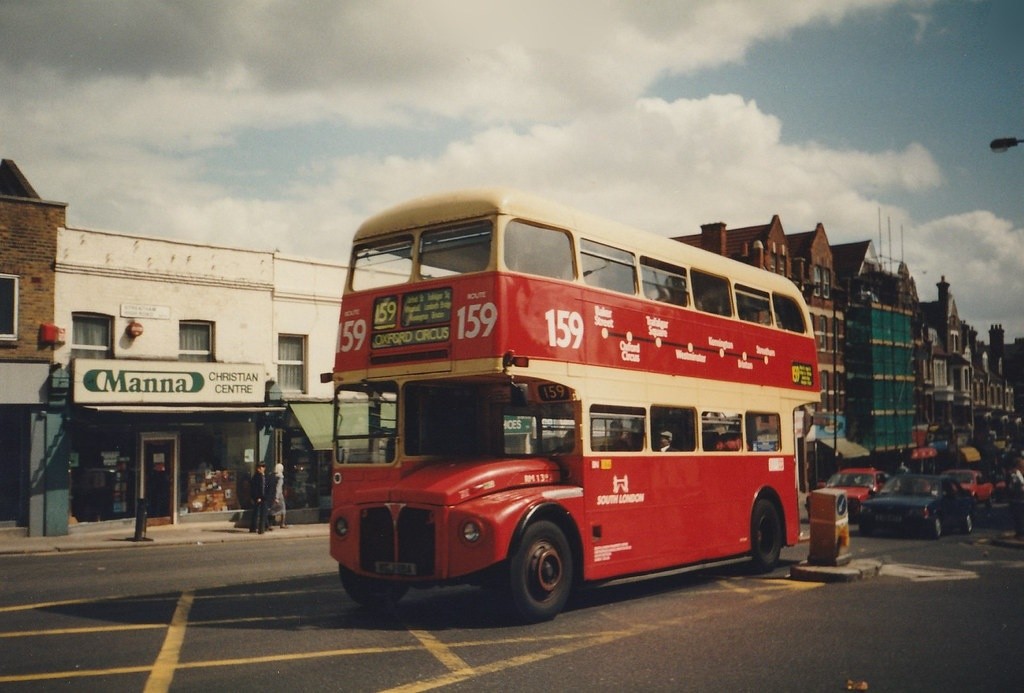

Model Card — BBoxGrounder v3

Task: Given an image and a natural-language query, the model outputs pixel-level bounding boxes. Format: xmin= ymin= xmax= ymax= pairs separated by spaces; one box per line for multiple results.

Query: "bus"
xmin=318 ymin=188 xmax=823 ymax=623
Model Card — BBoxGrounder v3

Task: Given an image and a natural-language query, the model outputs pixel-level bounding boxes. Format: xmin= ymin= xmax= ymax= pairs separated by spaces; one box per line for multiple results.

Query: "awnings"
xmin=288 ymin=401 xmax=396 ymax=449
xmin=960 ymin=447 xmax=981 ymax=462
xmin=820 ymin=439 xmax=870 ymax=458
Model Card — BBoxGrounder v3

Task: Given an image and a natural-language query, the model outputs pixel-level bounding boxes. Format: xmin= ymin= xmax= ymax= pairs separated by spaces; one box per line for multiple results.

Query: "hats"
xmin=256 ymin=461 xmax=267 ymax=467
xmin=659 ymin=431 xmax=672 ymax=441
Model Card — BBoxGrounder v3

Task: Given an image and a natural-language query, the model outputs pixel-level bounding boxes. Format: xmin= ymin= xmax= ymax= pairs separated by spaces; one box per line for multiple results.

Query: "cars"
xmin=805 ymin=467 xmax=894 ymax=525
xmin=942 ymin=469 xmax=996 ymax=510
xmin=983 ymin=454 xmax=1024 ymax=499
xmin=855 ymin=473 xmax=977 ymax=539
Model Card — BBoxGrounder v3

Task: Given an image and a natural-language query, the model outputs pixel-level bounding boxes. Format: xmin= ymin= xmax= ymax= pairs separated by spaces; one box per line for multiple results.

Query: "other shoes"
xmin=265 ymin=526 xmax=273 ymax=532
xmin=257 ymin=530 xmax=264 ymax=534
xmin=250 ymin=529 xmax=256 ymax=533
xmin=280 ymin=525 xmax=289 ymax=529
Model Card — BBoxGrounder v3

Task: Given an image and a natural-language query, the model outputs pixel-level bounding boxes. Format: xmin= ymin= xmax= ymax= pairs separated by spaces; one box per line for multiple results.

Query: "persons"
xmin=599 ymin=420 xmax=631 ymax=453
xmin=250 ymin=461 xmax=289 ymax=535
xmin=651 ymin=429 xmax=679 ymax=451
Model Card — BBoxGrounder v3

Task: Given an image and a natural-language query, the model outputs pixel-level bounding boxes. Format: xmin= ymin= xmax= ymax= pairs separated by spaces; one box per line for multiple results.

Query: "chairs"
xmin=712 ymin=434 xmax=741 ymax=451
xmin=751 ymin=308 xmax=771 ymax=325
